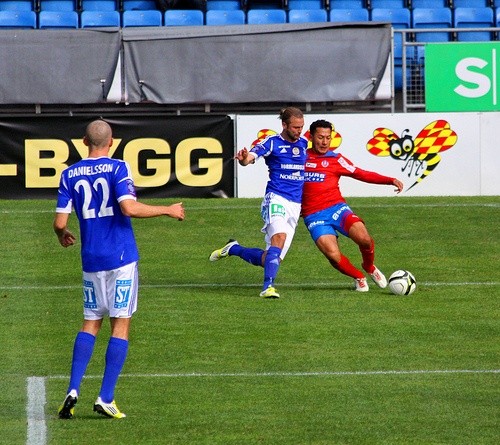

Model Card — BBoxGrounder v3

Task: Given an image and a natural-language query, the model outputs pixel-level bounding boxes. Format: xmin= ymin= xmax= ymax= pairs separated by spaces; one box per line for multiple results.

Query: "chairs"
xmin=0 ymin=0 xmax=500 ymax=87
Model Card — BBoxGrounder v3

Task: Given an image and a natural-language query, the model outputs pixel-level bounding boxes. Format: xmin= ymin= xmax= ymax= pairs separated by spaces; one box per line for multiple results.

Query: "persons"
xmin=54 ymin=119 xmax=185 ymax=419
xmin=301 ymin=119 xmax=403 ymax=292
xmin=208 ymin=107 xmax=308 ymax=298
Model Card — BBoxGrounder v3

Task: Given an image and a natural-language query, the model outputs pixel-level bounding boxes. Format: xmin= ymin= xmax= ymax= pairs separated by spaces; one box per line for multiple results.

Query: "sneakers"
xmin=360 ymin=263 xmax=388 ymax=288
xmin=208 ymin=238 xmax=240 ymax=262
xmin=94 ymin=396 xmax=125 ymax=418
xmin=258 ymin=286 xmax=280 ymax=299
xmin=354 ymin=277 xmax=369 ymax=292
xmin=57 ymin=389 xmax=78 ymax=419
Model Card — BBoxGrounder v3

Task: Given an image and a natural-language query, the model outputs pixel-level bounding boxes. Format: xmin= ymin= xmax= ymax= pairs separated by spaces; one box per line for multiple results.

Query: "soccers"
xmin=389 ymin=270 xmax=416 ymax=296
